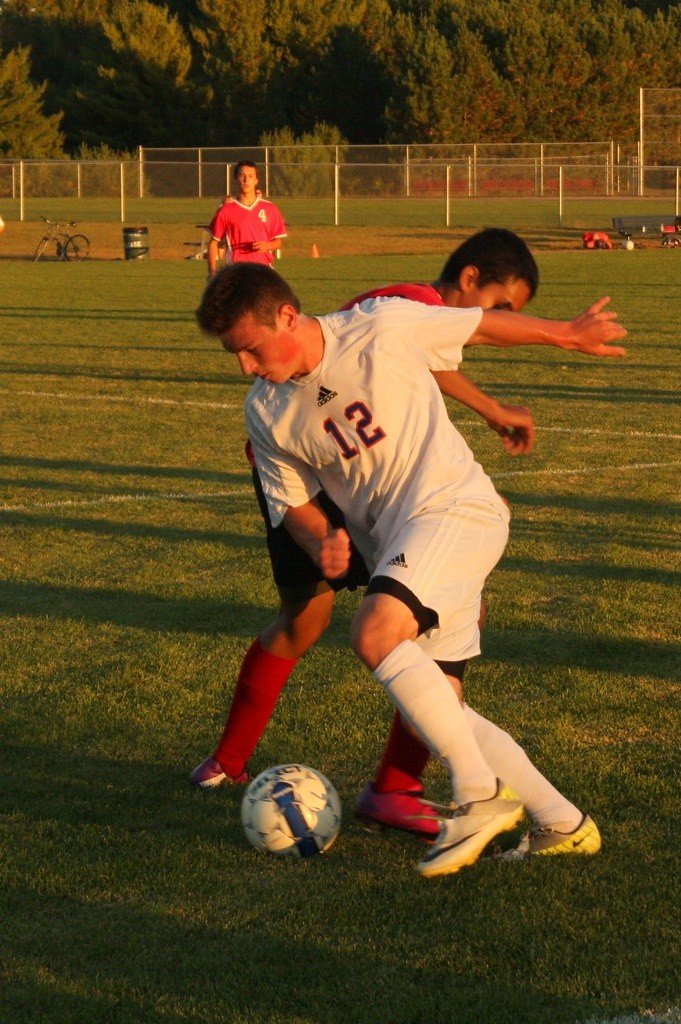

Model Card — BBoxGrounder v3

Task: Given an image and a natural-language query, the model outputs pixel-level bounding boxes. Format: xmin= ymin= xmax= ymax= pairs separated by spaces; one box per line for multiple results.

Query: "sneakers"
xmin=492 ymin=812 xmax=602 ymax=856
xmin=190 ymin=758 xmax=249 ymax=788
xmin=416 ymin=776 xmax=525 ymax=877
xmin=352 ymin=782 xmax=448 ymax=844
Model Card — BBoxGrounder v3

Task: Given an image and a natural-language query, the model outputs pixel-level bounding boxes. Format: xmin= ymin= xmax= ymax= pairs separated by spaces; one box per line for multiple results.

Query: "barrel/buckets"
xmin=123 ymin=228 xmax=150 ymax=261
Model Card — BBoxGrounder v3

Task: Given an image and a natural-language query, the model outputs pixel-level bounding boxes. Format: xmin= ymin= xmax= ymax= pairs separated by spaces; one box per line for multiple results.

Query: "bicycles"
xmin=28 ymin=215 xmax=93 ymax=263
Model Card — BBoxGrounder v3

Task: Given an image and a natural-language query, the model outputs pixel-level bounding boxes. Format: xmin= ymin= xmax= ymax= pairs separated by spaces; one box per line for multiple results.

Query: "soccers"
xmin=237 ymin=760 xmax=342 ymax=860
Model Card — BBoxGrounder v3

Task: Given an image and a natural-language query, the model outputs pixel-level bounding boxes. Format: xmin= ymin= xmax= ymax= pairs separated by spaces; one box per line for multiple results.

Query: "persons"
xmin=207 ymin=161 xmax=288 ymax=281
xmin=194 ymin=262 xmax=627 ymax=879
xmin=191 ymin=228 xmax=540 ymax=843
